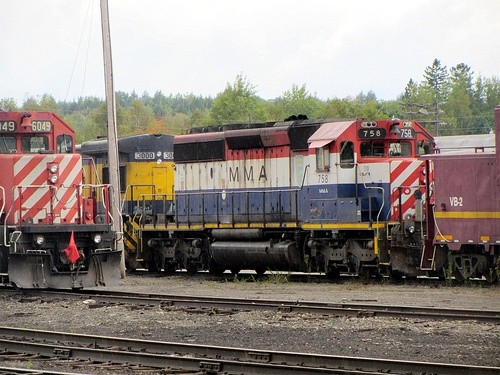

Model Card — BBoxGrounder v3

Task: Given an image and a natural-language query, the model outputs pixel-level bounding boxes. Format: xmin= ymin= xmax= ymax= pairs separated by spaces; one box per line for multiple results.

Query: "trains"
xmin=0 ymin=107 xmax=127 ymax=291
xmin=60 ymin=106 xmax=500 ymax=290
